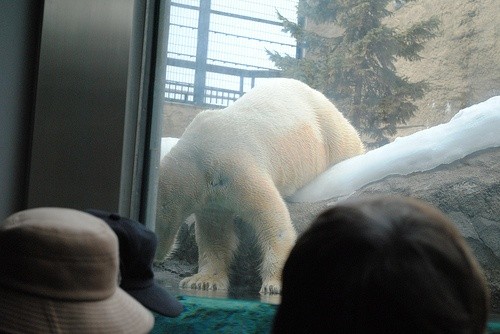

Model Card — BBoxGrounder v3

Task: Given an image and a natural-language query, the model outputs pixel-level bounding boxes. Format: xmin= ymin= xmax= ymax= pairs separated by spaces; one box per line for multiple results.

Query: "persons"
xmin=268 ymin=195 xmax=491 ymax=334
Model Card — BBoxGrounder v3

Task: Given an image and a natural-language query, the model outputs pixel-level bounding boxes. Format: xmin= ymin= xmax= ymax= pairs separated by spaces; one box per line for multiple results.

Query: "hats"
xmin=0 ymin=207 xmax=156 ymax=334
xmin=86 ymin=209 xmax=183 ymax=318
